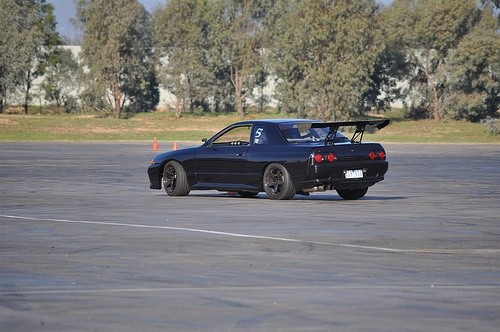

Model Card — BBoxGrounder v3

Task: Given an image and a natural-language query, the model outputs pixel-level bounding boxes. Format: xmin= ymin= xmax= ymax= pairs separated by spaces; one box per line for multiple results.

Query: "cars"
xmin=147 ymin=118 xmax=391 ymax=200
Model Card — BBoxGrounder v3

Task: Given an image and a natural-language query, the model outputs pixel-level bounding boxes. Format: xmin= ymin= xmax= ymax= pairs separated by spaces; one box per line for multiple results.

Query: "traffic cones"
xmin=152 ymin=135 xmax=159 ymax=151
xmin=173 ymin=142 xmax=178 ymax=150
